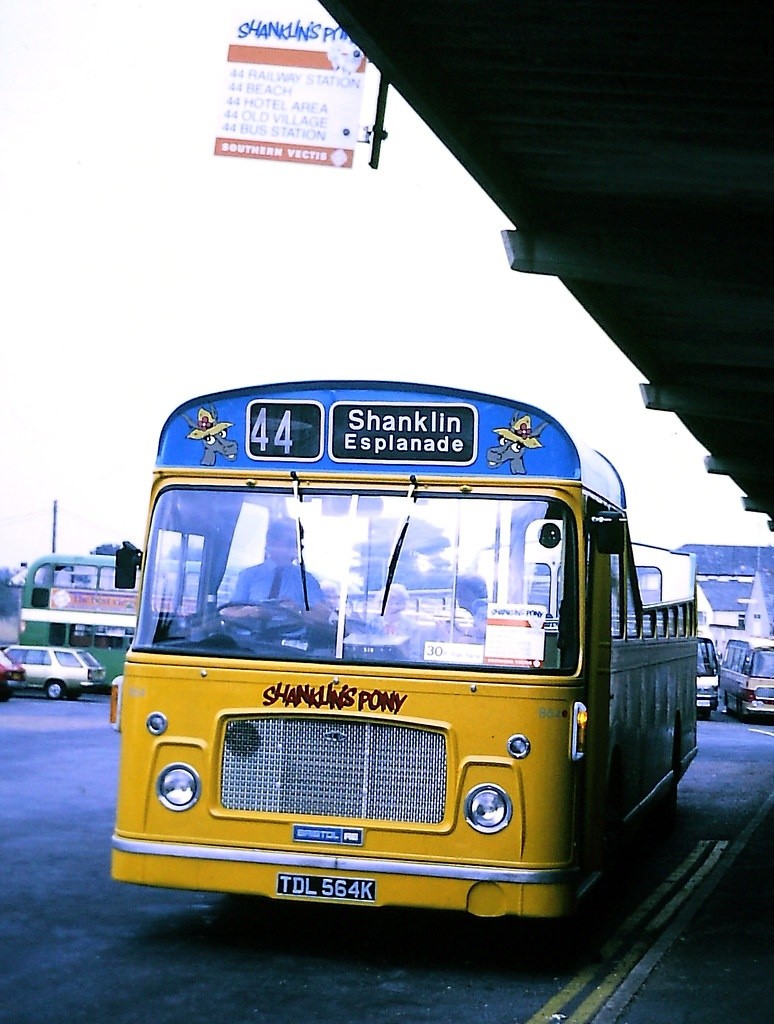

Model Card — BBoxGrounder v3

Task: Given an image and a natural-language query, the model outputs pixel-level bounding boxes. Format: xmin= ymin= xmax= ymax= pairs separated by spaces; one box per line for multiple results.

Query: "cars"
xmin=0 ymin=650 xmax=26 ymax=702
xmin=1 ymin=644 xmax=107 ymax=700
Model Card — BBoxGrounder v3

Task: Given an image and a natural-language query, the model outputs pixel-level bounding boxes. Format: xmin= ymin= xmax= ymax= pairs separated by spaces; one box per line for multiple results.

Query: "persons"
xmin=224 ymin=517 xmax=332 ymax=638
xmin=371 ymin=575 xmax=490 ymax=645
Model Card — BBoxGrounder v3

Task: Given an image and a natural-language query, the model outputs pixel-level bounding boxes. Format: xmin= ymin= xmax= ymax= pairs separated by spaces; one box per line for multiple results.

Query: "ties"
xmin=267 ymin=566 xmax=283 ymax=600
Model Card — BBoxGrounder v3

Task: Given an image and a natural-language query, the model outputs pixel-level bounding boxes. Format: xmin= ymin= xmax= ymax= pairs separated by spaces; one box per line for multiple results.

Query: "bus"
xmin=104 ymin=375 xmax=702 ymax=935
xmin=19 ymin=551 xmax=241 ymax=692
xmin=692 ymin=623 xmax=722 ymax=720
xmin=718 ymin=635 xmax=773 ymax=718
